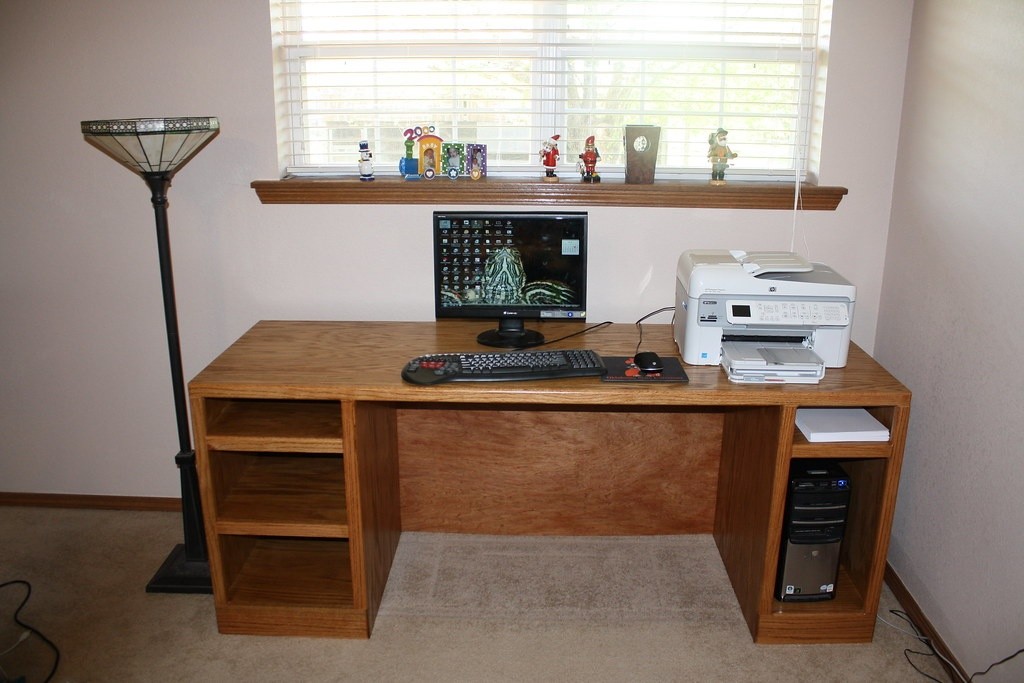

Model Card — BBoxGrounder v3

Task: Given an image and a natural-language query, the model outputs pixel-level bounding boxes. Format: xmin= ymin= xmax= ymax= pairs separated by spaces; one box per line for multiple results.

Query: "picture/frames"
xmin=417 ymin=134 xmax=444 ymax=175
xmin=441 ymin=142 xmax=464 ymax=175
xmin=466 ymin=143 xmax=487 ymax=177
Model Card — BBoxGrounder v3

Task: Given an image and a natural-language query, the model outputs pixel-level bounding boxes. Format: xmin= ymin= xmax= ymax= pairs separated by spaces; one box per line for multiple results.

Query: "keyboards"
xmin=403 ymin=350 xmax=607 ymax=384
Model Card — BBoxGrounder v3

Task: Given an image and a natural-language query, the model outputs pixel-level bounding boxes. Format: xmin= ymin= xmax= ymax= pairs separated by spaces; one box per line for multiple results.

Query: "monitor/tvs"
xmin=432 ymin=211 xmax=588 ymax=347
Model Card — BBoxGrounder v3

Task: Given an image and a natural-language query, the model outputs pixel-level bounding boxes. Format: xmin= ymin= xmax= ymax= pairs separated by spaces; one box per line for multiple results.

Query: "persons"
xmin=579 ymin=135 xmax=601 ymax=183
xmin=538 ymin=135 xmax=561 ymax=182
xmin=446 ymin=148 xmax=461 ymax=173
xmin=707 ymin=127 xmax=737 ymax=186
xmin=422 ymin=149 xmax=437 ymax=171
xmin=471 ymin=148 xmax=482 ymax=172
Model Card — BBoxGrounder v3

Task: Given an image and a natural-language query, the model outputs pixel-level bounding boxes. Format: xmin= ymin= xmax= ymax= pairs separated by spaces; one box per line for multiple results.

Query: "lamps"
xmin=80 ymin=116 xmax=219 ymax=594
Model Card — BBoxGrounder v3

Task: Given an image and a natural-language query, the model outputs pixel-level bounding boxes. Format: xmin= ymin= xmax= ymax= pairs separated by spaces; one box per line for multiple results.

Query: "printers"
xmin=672 ymin=249 xmax=857 ymax=384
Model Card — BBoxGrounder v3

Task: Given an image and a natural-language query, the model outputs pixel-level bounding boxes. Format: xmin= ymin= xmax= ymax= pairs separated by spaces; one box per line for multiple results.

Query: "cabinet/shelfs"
xmin=186 ymin=321 xmax=913 ymax=644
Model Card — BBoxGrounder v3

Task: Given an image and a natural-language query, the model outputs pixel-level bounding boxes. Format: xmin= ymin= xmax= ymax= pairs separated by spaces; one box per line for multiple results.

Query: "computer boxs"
xmin=775 ymin=457 xmax=852 ymax=602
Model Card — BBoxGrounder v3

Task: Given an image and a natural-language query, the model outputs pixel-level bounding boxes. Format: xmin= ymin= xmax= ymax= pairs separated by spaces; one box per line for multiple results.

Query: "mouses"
xmin=634 ymin=351 xmax=664 ymax=373
